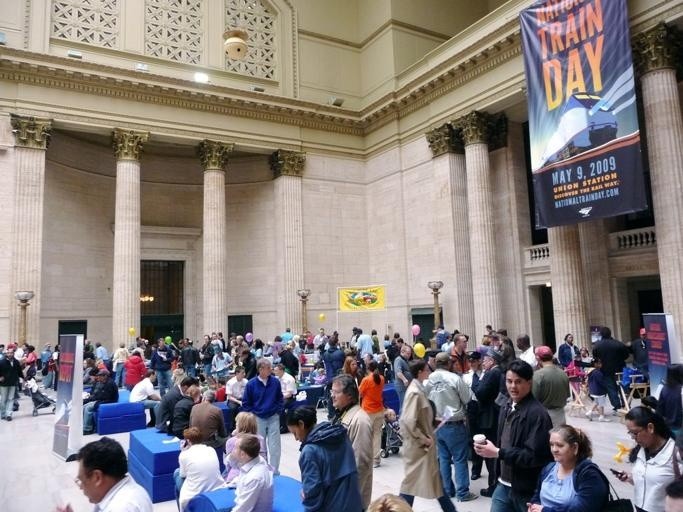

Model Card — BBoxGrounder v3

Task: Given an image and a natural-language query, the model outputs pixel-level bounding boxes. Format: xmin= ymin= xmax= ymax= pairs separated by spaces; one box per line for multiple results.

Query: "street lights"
xmin=295 ymin=288 xmax=314 ymax=354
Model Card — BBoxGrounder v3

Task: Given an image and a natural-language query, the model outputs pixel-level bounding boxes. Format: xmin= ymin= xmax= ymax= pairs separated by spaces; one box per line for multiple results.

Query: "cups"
xmin=473 ymin=432 xmax=486 ymax=445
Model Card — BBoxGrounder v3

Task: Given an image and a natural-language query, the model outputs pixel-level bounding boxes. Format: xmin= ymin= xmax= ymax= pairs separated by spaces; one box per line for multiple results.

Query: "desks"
xmin=221 ymin=26 xmax=250 ymax=63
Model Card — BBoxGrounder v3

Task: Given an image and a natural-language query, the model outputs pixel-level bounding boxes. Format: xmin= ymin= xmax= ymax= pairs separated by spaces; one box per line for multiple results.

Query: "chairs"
xmin=562 ymin=366 xmax=649 ymax=425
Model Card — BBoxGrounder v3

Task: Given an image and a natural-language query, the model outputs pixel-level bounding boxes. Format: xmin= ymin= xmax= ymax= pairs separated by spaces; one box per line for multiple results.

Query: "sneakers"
xmin=447 ymin=474 xmax=492 ymax=502
xmin=585 ymin=408 xmax=626 ymax=422
xmin=2 ymin=407 xmax=19 ymax=421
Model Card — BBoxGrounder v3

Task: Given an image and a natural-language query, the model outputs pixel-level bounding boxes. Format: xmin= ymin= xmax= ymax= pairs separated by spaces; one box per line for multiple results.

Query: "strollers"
xmin=379 ymin=401 xmax=403 ymax=458
xmin=22 ymin=375 xmax=56 ymax=416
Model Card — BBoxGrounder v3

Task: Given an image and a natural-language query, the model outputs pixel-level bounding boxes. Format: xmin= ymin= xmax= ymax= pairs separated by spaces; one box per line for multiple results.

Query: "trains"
xmin=536 ymin=89 xmax=618 ymax=169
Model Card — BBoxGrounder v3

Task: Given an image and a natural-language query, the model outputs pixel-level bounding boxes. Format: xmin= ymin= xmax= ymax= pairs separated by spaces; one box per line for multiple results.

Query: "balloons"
xmin=319 ymin=314 xmax=325 ymax=322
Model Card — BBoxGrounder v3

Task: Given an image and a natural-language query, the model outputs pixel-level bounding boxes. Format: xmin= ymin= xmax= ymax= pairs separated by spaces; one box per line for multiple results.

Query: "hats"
xmin=435 ymin=352 xmax=449 ymax=363
xmin=96 ymin=369 xmax=110 ymax=376
xmin=535 ymin=346 xmax=553 ymax=359
xmin=466 ymin=350 xmax=482 ymax=360
xmin=484 ymin=349 xmax=505 ymax=362
xmin=88 ymin=368 xmax=99 ymax=376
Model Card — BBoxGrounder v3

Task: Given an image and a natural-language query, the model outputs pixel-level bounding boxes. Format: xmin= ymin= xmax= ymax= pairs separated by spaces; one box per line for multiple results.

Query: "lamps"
xmin=0 ymin=31 xmax=7 ymax=46
xmin=295 ymin=289 xmax=312 ymax=338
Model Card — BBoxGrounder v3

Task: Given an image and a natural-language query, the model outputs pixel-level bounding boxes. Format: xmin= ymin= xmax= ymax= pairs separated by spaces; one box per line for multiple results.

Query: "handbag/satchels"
xmin=580 ymin=464 xmax=634 ymax=511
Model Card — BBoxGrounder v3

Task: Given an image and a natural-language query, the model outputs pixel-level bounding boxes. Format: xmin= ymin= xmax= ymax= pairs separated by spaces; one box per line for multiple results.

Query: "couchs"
xmin=186 ymin=476 xmax=305 ymax=512
xmin=298 ymin=383 xmax=327 ymax=408
xmin=213 ymin=402 xmax=231 ymax=431
xmin=94 ymin=389 xmax=146 ymax=436
xmin=383 ymin=382 xmax=399 ymax=413
xmin=128 ymin=426 xmax=223 ymax=504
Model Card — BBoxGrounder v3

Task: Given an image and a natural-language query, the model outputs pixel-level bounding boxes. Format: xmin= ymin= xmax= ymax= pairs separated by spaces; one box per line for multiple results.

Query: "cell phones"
xmin=610 ymin=468 xmax=628 ymax=478
